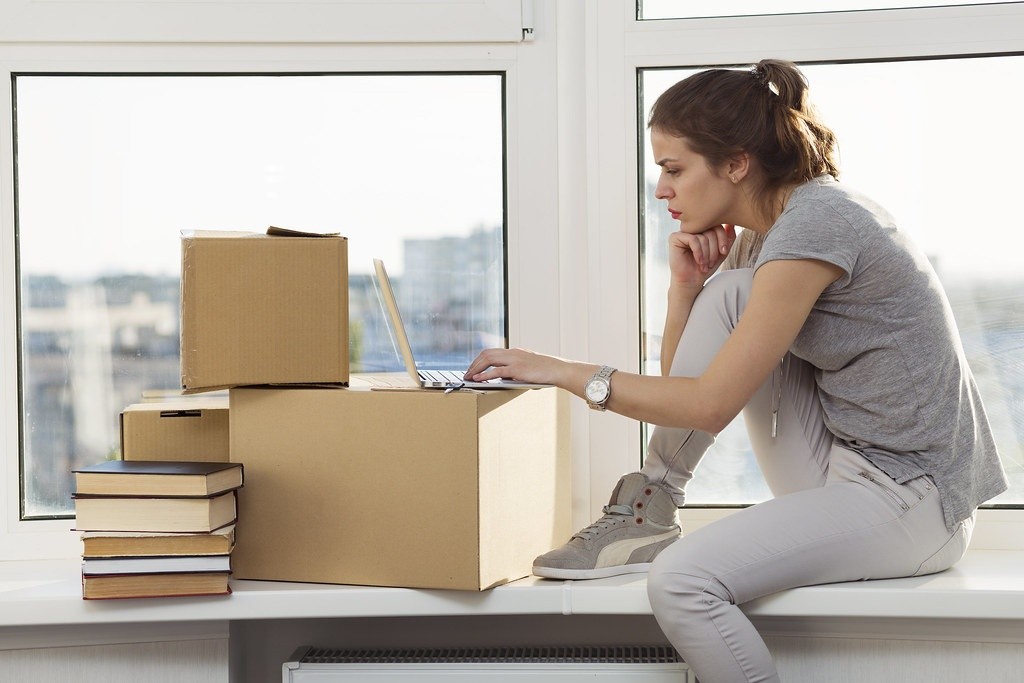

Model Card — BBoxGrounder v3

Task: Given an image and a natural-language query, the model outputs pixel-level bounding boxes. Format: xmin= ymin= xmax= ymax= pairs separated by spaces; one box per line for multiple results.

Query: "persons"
xmin=463 ymin=60 xmax=1009 ymax=683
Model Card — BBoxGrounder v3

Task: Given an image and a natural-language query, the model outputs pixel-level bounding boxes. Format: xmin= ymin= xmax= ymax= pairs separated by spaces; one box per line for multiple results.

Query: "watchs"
xmin=584 ymin=366 xmax=618 ymax=412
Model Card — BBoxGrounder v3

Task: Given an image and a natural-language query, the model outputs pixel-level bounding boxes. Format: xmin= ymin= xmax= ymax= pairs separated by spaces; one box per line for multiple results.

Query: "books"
xmin=71 ymin=460 xmax=245 ymax=496
xmin=82 ymin=571 xmax=233 ymax=600
xmin=82 ymin=553 xmax=233 ymax=576
xmin=70 ymin=489 xmax=239 ymax=534
xmin=80 ymin=524 xmax=236 ymax=557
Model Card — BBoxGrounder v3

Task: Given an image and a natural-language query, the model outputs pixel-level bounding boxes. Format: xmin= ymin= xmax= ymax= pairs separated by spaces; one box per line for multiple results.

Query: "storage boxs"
xmin=118 ymin=403 xmax=229 ymax=463
xmin=228 ymin=385 xmax=573 ymax=591
xmin=180 ymin=228 xmax=350 ymax=395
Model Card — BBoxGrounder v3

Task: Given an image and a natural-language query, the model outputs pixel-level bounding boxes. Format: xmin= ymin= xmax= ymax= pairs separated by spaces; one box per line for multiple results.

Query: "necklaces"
xmin=771 ymin=185 xmax=787 ymax=438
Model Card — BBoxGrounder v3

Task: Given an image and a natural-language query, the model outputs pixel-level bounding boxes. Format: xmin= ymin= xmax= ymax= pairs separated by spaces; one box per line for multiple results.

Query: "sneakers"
xmin=532 ymin=472 xmax=683 ymax=579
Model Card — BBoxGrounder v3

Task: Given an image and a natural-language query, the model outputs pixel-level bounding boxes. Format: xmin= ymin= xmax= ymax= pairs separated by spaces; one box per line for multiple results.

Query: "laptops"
xmin=374 ymin=259 xmax=555 ymax=389
